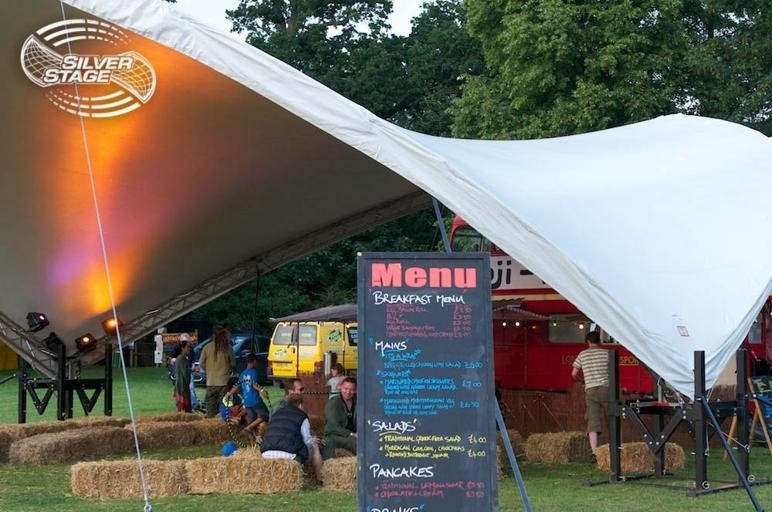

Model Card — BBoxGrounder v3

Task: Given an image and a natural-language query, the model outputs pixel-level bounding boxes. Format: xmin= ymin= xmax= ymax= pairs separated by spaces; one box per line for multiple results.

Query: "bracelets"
xmin=350 ymin=432 xmax=354 ymax=437
xmin=260 ymin=389 xmax=264 ymax=393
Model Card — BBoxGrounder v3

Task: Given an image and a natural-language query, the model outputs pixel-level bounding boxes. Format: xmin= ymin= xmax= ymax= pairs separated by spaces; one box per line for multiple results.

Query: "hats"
xmin=585 ymin=332 xmax=599 ymax=343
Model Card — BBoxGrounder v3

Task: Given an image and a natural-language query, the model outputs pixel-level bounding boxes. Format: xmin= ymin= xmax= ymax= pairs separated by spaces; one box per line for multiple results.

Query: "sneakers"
xmin=241 ymin=427 xmax=263 ymax=444
xmin=591 ymin=456 xmax=596 ymax=463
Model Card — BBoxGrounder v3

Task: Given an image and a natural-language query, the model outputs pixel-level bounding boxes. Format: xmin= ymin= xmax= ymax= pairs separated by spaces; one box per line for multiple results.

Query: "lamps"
xmin=26 ymin=311 xmax=124 ymax=351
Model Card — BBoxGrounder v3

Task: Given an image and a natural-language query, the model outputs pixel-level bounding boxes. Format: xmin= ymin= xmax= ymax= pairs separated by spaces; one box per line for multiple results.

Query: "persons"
xmin=570 ymin=331 xmax=610 ymax=465
xmin=220 ymin=377 xmax=247 ymax=425
xmin=198 ymin=325 xmax=237 ymax=419
xmin=320 ymin=376 xmax=359 ymax=460
xmin=173 ymin=341 xmax=200 ymax=414
xmin=225 ymin=355 xmax=271 ymax=447
xmin=169 ymin=332 xmax=200 ymax=408
xmin=324 ymin=362 xmax=349 ymax=400
xmin=259 ymin=394 xmax=325 ymax=486
xmin=269 ymin=376 xmax=307 ymax=423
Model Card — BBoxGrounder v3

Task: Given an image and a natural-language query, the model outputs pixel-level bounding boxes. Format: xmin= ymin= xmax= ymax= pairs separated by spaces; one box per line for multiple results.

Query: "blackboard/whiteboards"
xmin=356 ymin=252 xmax=500 ymax=512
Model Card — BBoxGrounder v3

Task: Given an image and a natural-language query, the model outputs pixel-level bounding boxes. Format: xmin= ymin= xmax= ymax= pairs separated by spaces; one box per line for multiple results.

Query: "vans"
xmin=266 ymin=321 xmax=358 ymax=380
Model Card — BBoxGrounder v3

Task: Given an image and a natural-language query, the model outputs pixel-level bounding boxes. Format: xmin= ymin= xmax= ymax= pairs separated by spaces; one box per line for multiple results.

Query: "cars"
xmin=168 ymin=333 xmax=270 ymax=387
